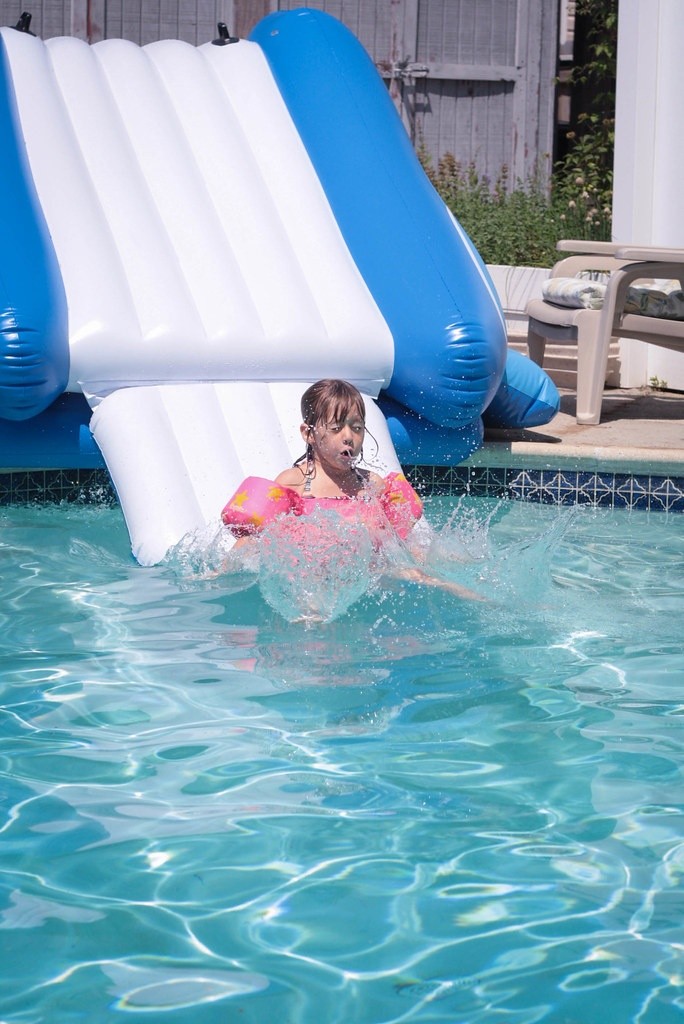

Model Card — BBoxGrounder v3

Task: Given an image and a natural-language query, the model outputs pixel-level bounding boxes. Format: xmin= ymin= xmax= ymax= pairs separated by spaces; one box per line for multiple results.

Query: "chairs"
xmin=524 ymin=240 xmax=684 ymax=425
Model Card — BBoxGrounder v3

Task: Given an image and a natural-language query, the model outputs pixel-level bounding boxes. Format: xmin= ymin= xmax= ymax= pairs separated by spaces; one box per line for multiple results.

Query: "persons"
xmin=222 ymin=378 xmax=482 ymax=605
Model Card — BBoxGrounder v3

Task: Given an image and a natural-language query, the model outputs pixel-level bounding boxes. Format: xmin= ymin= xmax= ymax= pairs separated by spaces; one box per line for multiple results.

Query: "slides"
xmin=0 ymin=5 xmax=507 ymax=580
